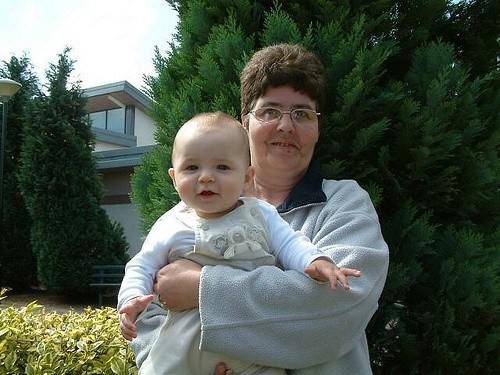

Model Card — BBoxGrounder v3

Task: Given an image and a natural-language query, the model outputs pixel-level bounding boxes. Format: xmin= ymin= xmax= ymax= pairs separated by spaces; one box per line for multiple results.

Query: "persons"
xmin=130 ymin=43 xmax=390 ymax=375
xmin=116 ymin=110 xmax=363 ymax=375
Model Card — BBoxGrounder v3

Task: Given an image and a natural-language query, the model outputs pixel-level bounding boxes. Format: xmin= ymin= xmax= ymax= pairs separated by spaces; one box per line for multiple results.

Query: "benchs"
xmin=90 ymin=265 xmax=125 ymax=305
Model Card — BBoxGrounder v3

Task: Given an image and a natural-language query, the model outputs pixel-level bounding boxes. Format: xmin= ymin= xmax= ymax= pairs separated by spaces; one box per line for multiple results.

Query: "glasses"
xmin=246 ymin=106 xmax=322 ymax=123
